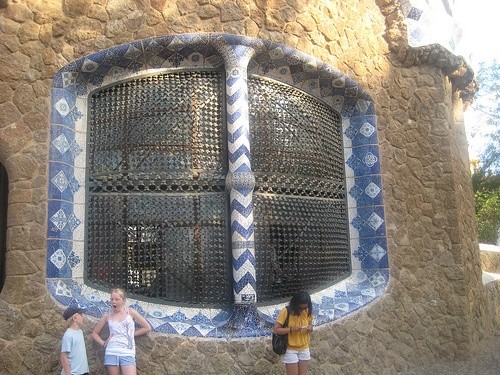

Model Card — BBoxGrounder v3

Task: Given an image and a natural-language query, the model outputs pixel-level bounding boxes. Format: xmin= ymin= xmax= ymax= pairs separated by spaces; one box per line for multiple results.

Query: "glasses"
xmin=298 ymin=307 xmax=308 ymax=310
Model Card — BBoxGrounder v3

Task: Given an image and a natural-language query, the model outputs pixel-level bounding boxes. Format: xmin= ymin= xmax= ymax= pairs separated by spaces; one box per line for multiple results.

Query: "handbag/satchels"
xmin=272 ymin=307 xmax=290 ymax=355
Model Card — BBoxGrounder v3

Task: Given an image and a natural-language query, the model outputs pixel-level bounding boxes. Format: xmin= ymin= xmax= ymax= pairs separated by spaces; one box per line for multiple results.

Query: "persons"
xmin=60 ymin=306 xmax=91 ymax=375
xmin=92 ymin=289 xmax=151 ymax=375
xmin=274 ymin=291 xmax=313 ymax=375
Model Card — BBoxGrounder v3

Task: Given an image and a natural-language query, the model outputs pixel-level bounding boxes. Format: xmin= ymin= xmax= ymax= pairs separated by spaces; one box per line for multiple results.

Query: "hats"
xmin=63 ymin=306 xmax=87 ymax=320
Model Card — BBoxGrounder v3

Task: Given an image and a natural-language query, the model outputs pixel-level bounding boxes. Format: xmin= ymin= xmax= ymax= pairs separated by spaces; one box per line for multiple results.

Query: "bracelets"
xmin=289 ymin=327 xmax=292 ymax=332
